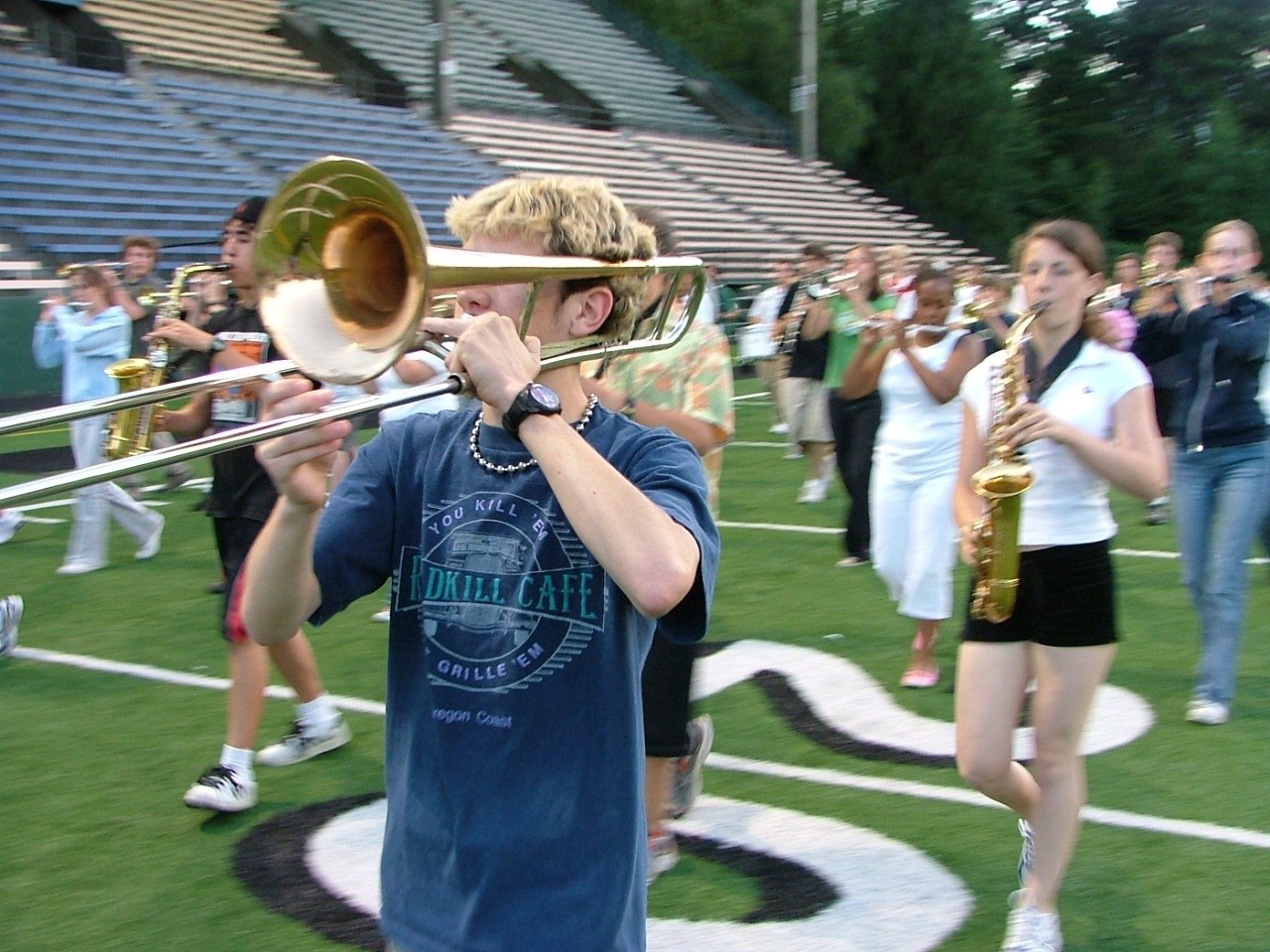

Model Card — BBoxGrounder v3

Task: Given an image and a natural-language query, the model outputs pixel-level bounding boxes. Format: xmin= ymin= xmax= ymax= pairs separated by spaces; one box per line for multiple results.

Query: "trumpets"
xmin=136 ymin=275 xmax=234 ymax=308
xmin=807 ymin=268 xmax=862 ymax=301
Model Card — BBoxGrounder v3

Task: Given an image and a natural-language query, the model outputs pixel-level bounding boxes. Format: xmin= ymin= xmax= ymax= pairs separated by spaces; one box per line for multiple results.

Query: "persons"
xmin=1 ymin=197 xmax=1270 ymax=814
xmin=950 ymin=222 xmax=1168 ymax=952
xmin=239 ymin=174 xmax=719 ymax=951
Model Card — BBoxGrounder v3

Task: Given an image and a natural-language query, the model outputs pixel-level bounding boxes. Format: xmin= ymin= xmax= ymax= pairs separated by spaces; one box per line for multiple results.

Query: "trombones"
xmin=0 ymin=157 xmax=704 ymax=511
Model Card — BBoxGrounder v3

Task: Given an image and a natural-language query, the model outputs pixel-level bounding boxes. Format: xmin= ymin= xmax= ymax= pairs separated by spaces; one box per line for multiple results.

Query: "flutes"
xmin=846 ymin=317 xmax=963 ymax=337
xmin=1156 ymin=272 xmax=1259 ymax=291
xmin=35 ymin=288 xmax=99 ymax=309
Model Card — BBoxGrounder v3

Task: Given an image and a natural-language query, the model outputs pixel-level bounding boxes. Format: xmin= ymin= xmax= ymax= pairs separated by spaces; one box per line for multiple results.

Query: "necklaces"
xmin=470 ymin=394 xmax=599 ymax=474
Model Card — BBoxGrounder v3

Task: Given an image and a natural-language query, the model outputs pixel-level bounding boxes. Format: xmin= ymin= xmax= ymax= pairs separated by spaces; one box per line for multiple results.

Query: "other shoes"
xmin=1184 ymin=698 xmax=1228 ymax=724
xmin=208 ymin=580 xmax=226 ymax=595
xmin=371 ymin=606 xmax=390 ymax=623
xmin=768 ymin=421 xmax=789 ymax=435
xmin=159 ymin=466 xmax=196 ymax=493
xmin=1142 ymin=502 xmax=1172 ymax=525
xmin=784 ymin=446 xmax=807 ymax=460
xmin=899 ymin=656 xmax=940 ymax=689
xmin=135 ymin=518 xmax=165 ymax=559
xmin=911 ymin=614 xmax=937 ymax=649
xmin=55 ymin=560 xmax=109 ymax=574
xmin=122 ymin=486 xmax=141 ymax=499
xmin=0 ymin=511 xmax=25 ymax=544
xmin=0 ymin=595 xmax=24 ymax=656
xmin=835 ymin=554 xmax=868 ymax=567
xmin=796 ymin=478 xmax=827 ymax=502
xmin=819 ymin=451 xmax=838 ymax=481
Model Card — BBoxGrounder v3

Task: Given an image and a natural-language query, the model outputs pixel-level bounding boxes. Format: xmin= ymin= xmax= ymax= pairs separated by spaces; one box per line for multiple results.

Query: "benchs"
xmin=0 ymin=1 xmax=1021 ymax=290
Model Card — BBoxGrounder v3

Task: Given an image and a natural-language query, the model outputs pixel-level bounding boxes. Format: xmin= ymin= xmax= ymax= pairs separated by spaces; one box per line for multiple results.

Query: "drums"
xmin=736 ymin=323 xmax=785 ymax=362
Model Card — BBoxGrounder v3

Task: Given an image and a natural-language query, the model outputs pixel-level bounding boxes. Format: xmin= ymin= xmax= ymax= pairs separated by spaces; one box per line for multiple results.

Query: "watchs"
xmin=502 ymin=383 xmax=563 ymax=441
xmin=206 ymin=337 xmax=226 ymax=355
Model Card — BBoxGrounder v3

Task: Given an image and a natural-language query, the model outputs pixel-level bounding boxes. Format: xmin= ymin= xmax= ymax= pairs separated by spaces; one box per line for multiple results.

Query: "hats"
xmin=232 ymin=195 xmax=272 ymax=223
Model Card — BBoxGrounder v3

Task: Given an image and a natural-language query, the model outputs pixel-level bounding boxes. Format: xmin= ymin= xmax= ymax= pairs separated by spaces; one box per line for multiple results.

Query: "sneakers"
xmin=671 ymin=715 xmax=713 ymax=821
xmin=256 ymin=711 xmax=353 ymax=767
xmin=1001 ymin=888 xmax=1062 ymax=952
xmin=184 ymin=765 xmax=259 ymax=810
xmin=642 ymin=829 xmax=680 ymax=887
xmin=1017 ymin=817 xmax=1036 ymax=889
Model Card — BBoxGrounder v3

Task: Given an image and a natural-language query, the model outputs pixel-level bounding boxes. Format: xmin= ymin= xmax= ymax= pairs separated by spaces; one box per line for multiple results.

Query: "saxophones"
xmin=96 ymin=261 xmax=240 ymax=458
xmin=971 ymin=299 xmax=1052 ymax=623
